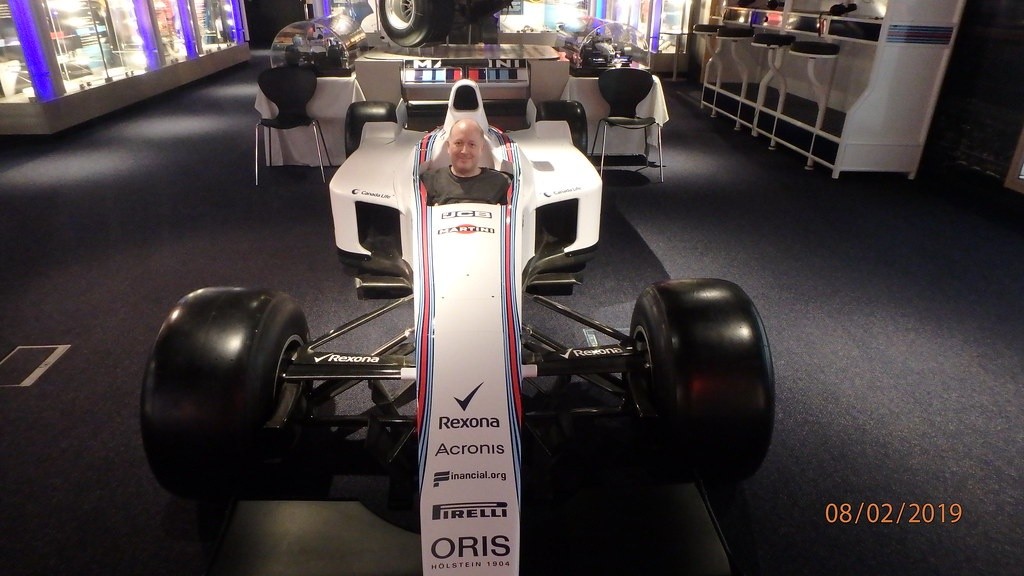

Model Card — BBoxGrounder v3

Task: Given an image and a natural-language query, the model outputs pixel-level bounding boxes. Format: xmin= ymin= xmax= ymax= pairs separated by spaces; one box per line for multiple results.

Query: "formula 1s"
xmin=140 ymin=97 xmax=778 ymax=576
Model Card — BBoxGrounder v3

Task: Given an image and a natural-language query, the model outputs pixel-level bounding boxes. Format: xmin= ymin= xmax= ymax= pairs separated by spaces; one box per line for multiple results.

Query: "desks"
xmin=355 ymin=44 xmax=571 ymax=102
xmin=255 ymin=71 xmax=366 ymax=169
xmin=561 ymin=66 xmax=669 ymax=165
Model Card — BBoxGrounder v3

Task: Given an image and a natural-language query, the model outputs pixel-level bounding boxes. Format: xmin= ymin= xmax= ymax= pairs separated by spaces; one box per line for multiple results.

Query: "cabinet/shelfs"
xmin=584 ymin=0 xmax=691 ymax=80
xmin=0 ymin=0 xmax=252 ymax=136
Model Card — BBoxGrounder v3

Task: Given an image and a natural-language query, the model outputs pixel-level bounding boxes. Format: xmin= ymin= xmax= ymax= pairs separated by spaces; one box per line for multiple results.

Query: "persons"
xmin=419 ymin=117 xmax=512 ymax=210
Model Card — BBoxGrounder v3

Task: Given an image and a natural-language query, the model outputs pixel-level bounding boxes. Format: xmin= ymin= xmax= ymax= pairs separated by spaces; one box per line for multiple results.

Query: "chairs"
xmin=587 ymin=67 xmax=668 ymax=186
xmin=252 ymin=66 xmax=333 ymax=185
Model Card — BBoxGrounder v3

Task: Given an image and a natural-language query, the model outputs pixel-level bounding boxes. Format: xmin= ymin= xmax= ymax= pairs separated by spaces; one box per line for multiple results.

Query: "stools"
xmin=690 ymin=23 xmax=840 ymax=172
